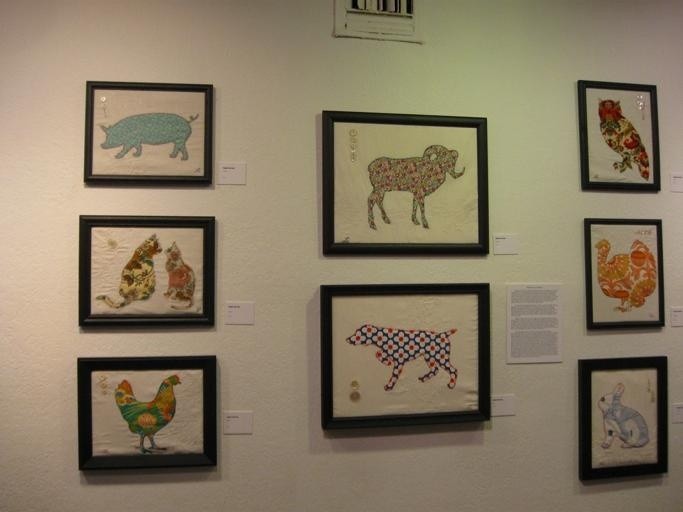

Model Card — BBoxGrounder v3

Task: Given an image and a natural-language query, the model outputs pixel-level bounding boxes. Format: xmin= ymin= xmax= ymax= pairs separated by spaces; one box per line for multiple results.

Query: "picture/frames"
xmin=577 ymin=353 xmax=670 ymax=482
xmin=316 ymin=281 xmax=494 ymax=432
xmin=577 ymin=78 xmax=661 ymax=193
xmin=84 ymin=80 xmax=214 ymax=191
xmin=320 ymin=109 xmax=489 ymax=257
xmin=582 ymin=216 xmax=667 ymax=330
xmin=78 ymin=214 xmax=216 ymax=329
xmin=75 ymin=355 xmax=219 ymax=471
